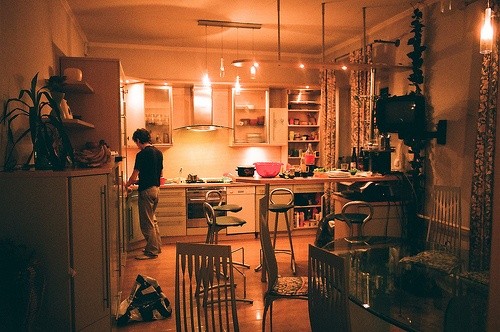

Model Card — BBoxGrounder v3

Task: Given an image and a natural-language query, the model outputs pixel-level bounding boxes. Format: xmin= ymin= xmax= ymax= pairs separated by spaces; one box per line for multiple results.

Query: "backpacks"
xmin=313 ymin=211 xmax=335 ymax=250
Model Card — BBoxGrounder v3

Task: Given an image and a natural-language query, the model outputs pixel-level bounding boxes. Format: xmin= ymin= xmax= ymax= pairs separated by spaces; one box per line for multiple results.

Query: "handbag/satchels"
xmin=116 ymin=273 xmax=172 ymax=322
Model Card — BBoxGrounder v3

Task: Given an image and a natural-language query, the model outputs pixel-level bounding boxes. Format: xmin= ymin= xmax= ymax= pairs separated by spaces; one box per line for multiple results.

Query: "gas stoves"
xmin=185 ymin=177 xmax=231 ymax=186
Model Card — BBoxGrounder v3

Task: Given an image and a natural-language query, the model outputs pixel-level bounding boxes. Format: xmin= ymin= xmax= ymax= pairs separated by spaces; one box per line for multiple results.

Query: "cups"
xmin=289 ymin=168 xmax=295 ymax=176
xmin=302 ymin=137 xmax=306 ymax=140
xmin=341 ymin=163 xmax=349 ymax=170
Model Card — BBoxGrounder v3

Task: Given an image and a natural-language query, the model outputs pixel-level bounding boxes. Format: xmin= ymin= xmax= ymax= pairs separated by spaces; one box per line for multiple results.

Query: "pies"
xmin=328 ymin=171 xmax=350 ymax=177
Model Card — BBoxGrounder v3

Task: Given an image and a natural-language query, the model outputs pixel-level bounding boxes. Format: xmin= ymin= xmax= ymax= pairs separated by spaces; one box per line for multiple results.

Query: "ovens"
xmin=186 ymin=186 xmax=226 ymax=235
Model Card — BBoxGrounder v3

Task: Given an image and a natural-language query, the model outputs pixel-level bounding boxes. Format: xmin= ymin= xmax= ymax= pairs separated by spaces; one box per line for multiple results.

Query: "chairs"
xmin=308 ymin=243 xmax=351 ymax=332
xmin=397 ymin=184 xmax=462 ymax=331
xmin=174 ymin=241 xmax=239 ymax=332
xmin=259 ymin=196 xmax=327 ymax=332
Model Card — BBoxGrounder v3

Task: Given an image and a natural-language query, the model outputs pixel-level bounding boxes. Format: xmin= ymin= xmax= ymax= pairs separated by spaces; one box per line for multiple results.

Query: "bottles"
xmin=350 ymin=147 xmax=357 ymax=170
xmin=305 ymin=143 xmax=315 ymax=176
xmin=358 ymin=146 xmax=364 ymax=170
xmin=301 ymin=153 xmax=305 ymax=173
xmin=295 ymin=119 xmax=299 ymax=125
xmin=163 ymin=133 xmax=169 ymax=144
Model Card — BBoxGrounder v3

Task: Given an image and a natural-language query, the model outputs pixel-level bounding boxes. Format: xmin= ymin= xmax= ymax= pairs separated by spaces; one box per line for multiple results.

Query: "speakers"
xmin=390 ymin=141 xmax=405 ymax=172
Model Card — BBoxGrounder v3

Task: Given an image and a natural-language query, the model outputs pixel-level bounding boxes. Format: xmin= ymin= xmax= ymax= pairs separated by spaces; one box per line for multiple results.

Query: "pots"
xmin=188 ymin=173 xmax=199 ymax=180
xmin=236 ymin=166 xmax=256 ymax=177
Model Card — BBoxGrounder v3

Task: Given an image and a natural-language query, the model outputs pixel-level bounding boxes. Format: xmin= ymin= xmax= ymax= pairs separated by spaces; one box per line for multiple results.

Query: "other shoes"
xmin=136 ymin=250 xmax=158 ymax=259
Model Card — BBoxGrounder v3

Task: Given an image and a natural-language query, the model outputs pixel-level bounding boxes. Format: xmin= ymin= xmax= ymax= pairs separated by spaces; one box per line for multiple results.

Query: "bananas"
xmin=77 ymin=140 xmax=111 ymax=167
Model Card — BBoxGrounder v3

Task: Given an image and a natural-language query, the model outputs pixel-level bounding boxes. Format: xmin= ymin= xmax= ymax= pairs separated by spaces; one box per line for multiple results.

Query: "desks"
xmin=322 ymin=235 xmax=490 ymax=332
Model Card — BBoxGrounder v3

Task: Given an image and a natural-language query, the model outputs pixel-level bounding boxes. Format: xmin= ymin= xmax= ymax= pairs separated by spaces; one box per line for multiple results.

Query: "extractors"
xmin=173 ymin=85 xmax=233 ymax=132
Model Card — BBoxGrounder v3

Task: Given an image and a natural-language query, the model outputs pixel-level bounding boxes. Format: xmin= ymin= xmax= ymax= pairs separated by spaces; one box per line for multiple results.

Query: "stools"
xmin=335 ymin=201 xmax=374 ymax=250
xmin=195 ymin=183 xmax=297 ymax=305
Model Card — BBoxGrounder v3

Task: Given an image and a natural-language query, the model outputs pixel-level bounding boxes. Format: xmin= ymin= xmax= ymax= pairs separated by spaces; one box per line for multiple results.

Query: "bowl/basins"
xmin=253 ymin=162 xmax=284 ymax=178
xmin=239 ymin=116 xmax=265 ymax=143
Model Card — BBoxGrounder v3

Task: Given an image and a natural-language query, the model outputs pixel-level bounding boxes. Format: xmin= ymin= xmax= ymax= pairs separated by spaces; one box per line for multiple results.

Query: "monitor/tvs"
xmin=379 ymin=95 xmax=427 ymax=134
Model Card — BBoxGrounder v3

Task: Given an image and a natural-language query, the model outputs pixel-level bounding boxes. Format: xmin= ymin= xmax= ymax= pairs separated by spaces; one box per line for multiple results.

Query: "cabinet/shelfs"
xmin=126 ymin=179 xmax=324 ymax=244
xmin=0 ymin=54 xmax=128 ymax=332
xmin=228 ymin=88 xmax=323 ymax=171
xmin=125 ymin=83 xmax=173 ymax=149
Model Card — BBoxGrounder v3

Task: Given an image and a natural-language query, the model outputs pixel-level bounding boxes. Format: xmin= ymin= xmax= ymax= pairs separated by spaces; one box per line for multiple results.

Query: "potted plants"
xmin=4 ymin=71 xmax=83 ymax=172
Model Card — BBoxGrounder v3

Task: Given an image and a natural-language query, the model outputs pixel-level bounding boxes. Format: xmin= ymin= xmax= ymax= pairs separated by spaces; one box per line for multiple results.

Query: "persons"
xmin=126 ymin=128 xmax=163 ymax=259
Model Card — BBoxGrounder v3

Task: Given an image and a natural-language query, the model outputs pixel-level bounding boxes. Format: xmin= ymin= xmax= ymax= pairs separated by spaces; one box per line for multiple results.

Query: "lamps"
xmin=197 ymin=20 xmax=263 ymax=95
xmin=478 ymin=0 xmax=493 ymax=54
